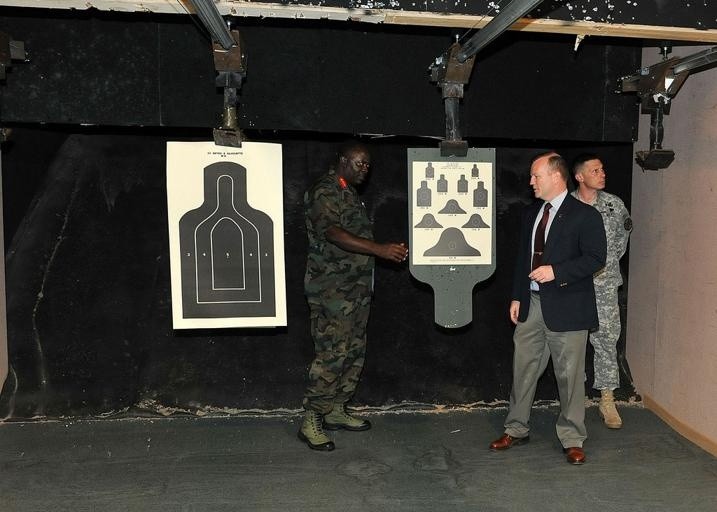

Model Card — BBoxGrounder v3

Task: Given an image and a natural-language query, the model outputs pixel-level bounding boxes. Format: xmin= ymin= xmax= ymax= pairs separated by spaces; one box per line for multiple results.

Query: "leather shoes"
xmin=564 ymin=446 xmax=586 ymax=466
xmin=490 ymin=433 xmax=530 ymax=450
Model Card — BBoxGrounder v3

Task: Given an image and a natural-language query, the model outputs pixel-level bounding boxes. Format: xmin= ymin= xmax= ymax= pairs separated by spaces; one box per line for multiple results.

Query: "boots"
xmin=297 ymin=410 xmax=335 ymax=452
xmin=322 ymin=401 xmax=371 ymax=432
xmin=597 ymin=390 xmax=623 ymax=429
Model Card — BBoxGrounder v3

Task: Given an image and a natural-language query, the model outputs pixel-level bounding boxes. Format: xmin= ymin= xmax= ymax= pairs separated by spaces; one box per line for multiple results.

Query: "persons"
xmin=487 ymin=153 xmax=608 ymax=465
xmin=295 ymin=133 xmax=409 ymax=451
xmin=569 ymin=153 xmax=633 ymax=428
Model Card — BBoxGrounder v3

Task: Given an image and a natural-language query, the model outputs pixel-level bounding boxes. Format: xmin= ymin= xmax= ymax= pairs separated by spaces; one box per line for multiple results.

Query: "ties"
xmin=532 ymin=202 xmax=552 ymax=285
